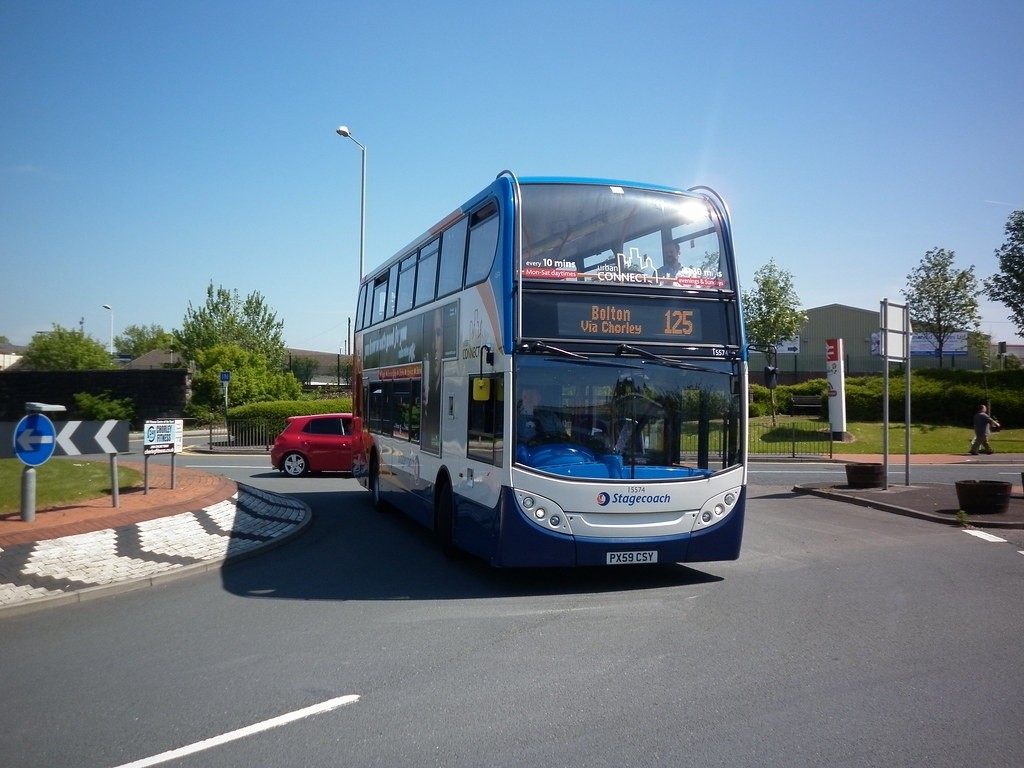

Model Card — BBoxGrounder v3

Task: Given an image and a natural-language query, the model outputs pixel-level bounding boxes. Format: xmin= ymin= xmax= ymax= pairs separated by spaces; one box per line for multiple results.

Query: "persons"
xmin=653 ymin=242 xmax=684 ymax=285
xmin=519 ymin=385 xmax=567 ymax=445
xmin=971 ymin=405 xmax=1000 ymax=455
xmin=424 ymin=309 xmax=441 ymax=452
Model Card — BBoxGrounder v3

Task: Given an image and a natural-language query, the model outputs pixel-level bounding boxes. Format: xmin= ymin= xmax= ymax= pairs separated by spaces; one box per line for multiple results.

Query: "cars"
xmin=270 ymin=412 xmax=354 ymax=477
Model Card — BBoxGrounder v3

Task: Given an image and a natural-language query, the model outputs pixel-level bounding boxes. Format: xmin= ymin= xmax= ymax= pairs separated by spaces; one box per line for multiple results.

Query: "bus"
xmin=352 ymin=169 xmax=779 ymax=572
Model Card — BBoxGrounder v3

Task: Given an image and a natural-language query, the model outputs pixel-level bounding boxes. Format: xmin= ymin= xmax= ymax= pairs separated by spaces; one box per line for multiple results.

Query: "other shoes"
xmin=972 ymin=453 xmax=979 ymax=455
xmin=987 ymin=451 xmax=994 ymax=454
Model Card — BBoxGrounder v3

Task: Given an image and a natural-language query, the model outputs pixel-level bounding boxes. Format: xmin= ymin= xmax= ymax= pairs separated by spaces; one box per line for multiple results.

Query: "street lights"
xmin=335 ymin=126 xmax=366 ymax=285
xmin=103 ymin=304 xmax=113 ymax=355
